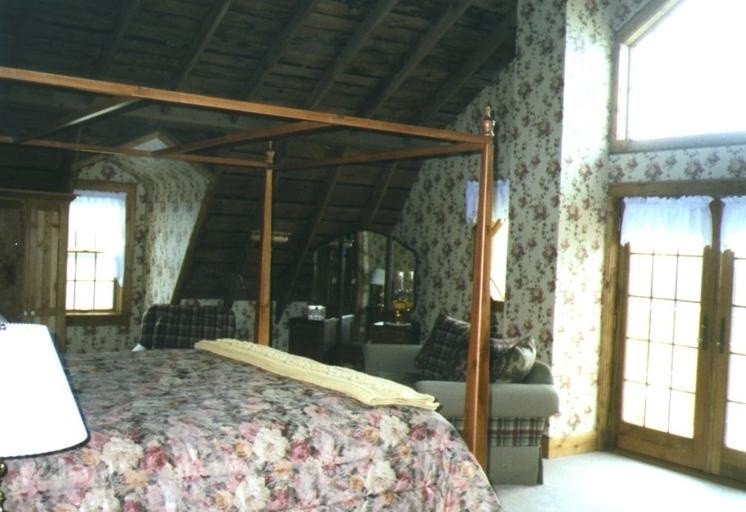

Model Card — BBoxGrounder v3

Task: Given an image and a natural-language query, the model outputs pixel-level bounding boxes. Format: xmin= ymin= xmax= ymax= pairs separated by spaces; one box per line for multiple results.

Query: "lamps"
xmin=1 ymin=323 xmax=88 ymax=512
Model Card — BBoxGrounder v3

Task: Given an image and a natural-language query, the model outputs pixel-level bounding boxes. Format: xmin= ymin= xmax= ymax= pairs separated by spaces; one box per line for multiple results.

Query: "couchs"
xmin=359 ymin=343 xmax=558 ymax=486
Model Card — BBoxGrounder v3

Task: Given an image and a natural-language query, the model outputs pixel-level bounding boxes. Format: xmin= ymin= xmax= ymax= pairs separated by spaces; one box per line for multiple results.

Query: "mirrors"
xmin=313 ymin=230 xmax=417 ymax=319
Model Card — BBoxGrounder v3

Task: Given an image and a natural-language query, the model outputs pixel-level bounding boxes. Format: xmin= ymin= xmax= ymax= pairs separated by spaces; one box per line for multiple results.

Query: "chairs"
xmin=140 ymin=303 xmax=235 ymax=351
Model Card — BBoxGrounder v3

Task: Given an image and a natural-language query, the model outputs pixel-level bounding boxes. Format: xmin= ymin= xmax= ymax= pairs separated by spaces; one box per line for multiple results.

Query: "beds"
xmin=0 ymin=351 xmax=460 ymax=512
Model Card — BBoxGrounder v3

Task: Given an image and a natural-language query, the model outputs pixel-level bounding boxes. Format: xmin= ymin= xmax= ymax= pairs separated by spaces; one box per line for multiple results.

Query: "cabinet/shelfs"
xmin=1 ymin=188 xmax=80 ymax=353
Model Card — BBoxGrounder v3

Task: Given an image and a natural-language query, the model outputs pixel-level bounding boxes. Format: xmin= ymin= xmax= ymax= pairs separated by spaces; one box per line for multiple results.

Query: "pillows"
xmin=411 ymin=312 xmax=536 ymax=383
